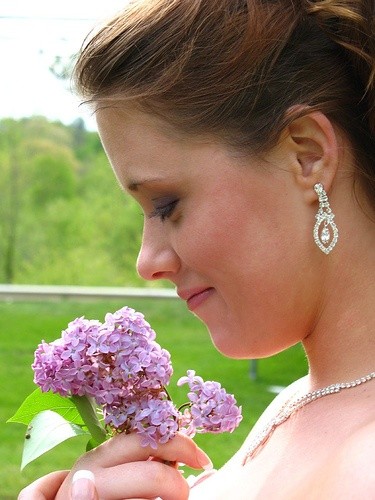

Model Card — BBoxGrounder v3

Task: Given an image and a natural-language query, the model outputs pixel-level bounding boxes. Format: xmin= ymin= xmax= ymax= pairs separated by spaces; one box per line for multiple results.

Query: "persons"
xmin=20 ymin=0 xmax=374 ymax=500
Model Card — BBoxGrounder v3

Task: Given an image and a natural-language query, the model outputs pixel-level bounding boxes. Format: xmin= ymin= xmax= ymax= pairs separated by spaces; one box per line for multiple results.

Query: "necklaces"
xmin=241 ymin=368 xmax=374 ymax=466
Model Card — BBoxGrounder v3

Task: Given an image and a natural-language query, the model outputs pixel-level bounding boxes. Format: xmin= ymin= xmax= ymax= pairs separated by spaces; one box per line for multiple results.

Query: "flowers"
xmin=5 ymin=306 xmax=243 ymax=467
